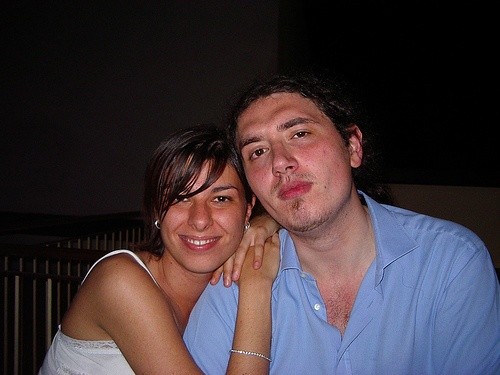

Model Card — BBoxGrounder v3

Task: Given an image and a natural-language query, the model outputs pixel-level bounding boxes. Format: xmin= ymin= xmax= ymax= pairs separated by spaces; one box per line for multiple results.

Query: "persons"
xmin=38 ymin=126 xmax=285 ymax=375
xmin=183 ymin=72 xmax=500 ymax=375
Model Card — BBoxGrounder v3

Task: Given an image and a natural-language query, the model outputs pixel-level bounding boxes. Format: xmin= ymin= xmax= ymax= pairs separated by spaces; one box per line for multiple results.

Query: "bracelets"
xmin=229 ymin=349 xmax=272 ymax=362
